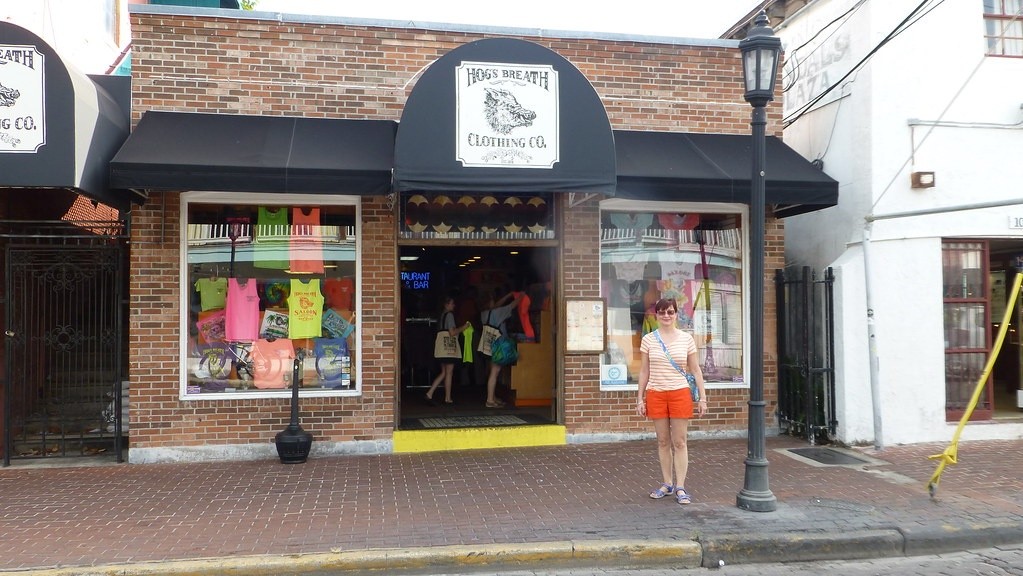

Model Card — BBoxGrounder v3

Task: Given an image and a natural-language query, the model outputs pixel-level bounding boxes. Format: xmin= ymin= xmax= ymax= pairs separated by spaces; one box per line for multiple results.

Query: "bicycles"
xmin=208 ymin=337 xmax=282 ymax=384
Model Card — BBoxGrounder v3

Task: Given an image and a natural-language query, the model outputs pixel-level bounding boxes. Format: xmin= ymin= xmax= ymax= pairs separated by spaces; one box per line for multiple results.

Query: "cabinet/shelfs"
xmin=496 ymin=281 xmax=555 ymax=405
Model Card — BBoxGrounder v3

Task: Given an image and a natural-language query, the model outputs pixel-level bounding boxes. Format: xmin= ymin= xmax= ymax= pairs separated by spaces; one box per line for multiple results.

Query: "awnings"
xmin=613 ymin=130 xmax=838 ymax=219
xmin=109 ymin=109 xmax=399 ymax=195
xmin=0 ymin=20 xmax=130 ymax=210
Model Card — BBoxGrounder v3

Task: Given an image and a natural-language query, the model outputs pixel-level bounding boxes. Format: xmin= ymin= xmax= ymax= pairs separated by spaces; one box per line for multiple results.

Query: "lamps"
xmin=510 ymin=248 xmax=518 ymax=254
xmin=457 ymin=252 xmax=481 ymax=267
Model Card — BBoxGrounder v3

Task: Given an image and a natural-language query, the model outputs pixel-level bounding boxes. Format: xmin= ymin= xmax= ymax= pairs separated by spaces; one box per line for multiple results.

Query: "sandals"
xmin=649 ymin=483 xmax=674 ymax=498
xmin=675 ymin=487 xmax=691 ymax=504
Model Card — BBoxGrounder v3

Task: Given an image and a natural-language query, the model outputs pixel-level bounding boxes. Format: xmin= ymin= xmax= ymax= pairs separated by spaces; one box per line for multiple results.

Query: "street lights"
xmin=735 ymin=8 xmax=784 ymax=512
xmin=227 ymin=215 xmax=240 ymax=365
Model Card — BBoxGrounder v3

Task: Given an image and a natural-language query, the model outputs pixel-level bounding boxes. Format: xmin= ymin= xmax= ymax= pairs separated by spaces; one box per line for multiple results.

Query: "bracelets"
xmin=637 ymin=402 xmax=641 ymax=405
xmin=699 ymin=400 xmax=707 ymax=402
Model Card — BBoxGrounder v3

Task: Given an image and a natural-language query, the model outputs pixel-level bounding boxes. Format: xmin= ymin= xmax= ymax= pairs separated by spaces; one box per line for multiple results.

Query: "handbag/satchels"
xmin=687 ymin=374 xmax=700 ymax=402
xmin=476 ymin=309 xmax=502 ymax=357
xmin=433 ymin=310 xmax=463 ymax=362
xmin=490 ymin=335 xmax=520 ymax=366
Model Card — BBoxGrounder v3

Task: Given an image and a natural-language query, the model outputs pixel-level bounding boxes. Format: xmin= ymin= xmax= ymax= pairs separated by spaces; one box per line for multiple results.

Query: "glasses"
xmin=657 ymin=309 xmax=674 ymax=316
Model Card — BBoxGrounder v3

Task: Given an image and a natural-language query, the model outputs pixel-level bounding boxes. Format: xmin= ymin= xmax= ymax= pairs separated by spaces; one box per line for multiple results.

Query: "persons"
xmin=425 ymin=297 xmax=470 ymax=405
xmin=455 ymin=285 xmax=487 ymax=404
xmin=478 ymin=289 xmax=528 ymax=409
xmin=636 ymin=297 xmax=709 ymax=505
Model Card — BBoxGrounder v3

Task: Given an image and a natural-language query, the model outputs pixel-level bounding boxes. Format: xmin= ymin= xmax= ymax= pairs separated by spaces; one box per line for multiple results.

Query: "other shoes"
xmin=495 ymin=399 xmax=507 ymax=406
xmin=485 ymin=401 xmax=504 ymax=408
xmin=424 ymin=393 xmax=432 ymax=401
xmin=445 ymin=400 xmax=453 ymax=404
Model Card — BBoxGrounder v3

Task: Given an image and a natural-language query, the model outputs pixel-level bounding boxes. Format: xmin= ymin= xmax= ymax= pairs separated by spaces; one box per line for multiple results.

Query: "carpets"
xmin=400 ymin=414 xmax=557 ymax=431
xmin=418 ymin=415 xmax=527 ymax=427
